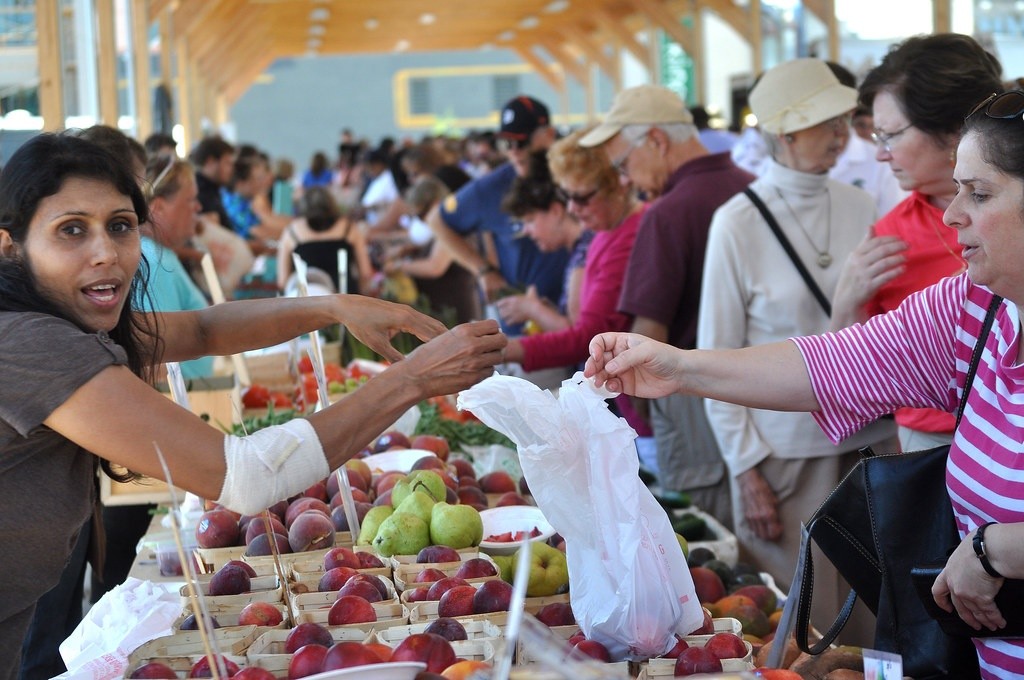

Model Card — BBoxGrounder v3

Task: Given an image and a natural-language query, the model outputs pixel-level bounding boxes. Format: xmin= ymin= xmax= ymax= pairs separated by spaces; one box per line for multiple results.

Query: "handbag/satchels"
xmin=796 ymin=290 xmax=1009 ymax=680
xmin=455 ymin=372 xmax=703 ymax=661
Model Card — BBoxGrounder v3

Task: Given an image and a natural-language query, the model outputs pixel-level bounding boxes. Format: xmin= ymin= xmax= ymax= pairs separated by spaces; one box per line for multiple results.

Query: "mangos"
xmin=687 ymin=544 xmax=866 ymax=679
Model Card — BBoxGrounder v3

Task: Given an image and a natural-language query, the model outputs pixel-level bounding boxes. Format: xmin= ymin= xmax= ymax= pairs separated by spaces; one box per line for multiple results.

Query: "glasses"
xmin=503 ymin=130 xmax=539 ymax=151
xmin=614 ymin=134 xmax=645 ymax=176
xmin=559 ymin=184 xmax=607 ymax=206
xmin=963 ymin=89 xmax=1024 ymax=123
xmin=872 ymin=119 xmax=919 ymax=152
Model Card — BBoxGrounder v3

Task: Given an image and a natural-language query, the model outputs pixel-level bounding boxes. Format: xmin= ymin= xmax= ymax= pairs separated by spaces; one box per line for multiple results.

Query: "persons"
xmin=62 ymin=60 xmax=914 ymax=605
xmin=0 ymin=132 xmax=508 ymax=680
xmin=829 ymin=33 xmax=1005 ymax=453
xmin=583 ymin=90 xmax=1024 ymax=680
xmin=697 ymin=57 xmax=902 ymax=650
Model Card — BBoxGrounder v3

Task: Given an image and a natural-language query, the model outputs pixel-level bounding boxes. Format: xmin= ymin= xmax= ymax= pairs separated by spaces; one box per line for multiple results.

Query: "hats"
xmin=578 ymin=84 xmax=694 ymax=147
xmin=751 ymin=56 xmax=861 ymax=135
xmin=495 ymin=97 xmax=549 ymax=140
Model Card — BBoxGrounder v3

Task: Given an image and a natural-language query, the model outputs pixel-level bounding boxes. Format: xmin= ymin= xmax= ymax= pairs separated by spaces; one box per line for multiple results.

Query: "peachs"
xmin=127 ymin=545 xmax=747 ymax=680
xmin=191 ymin=432 xmax=536 ymax=557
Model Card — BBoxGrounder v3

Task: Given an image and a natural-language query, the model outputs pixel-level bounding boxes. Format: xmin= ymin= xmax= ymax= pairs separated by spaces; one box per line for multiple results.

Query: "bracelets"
xmin=972 ymin=522 xmax=1002 ymax=578
xmin=477 ymin=266 xmax=494 ymax=279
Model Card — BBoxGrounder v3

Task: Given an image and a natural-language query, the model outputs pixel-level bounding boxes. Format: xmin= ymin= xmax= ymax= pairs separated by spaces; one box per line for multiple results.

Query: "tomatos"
xmin=240 ymin=353 xmax=479 ymax=422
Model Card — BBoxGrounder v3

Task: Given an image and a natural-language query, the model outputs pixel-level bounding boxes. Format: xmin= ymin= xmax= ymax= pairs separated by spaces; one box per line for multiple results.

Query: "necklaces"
xmin=776 ymin=187 xmax=832 ymax=270
xmin=928 ymin=212 xmax=968 ymax=277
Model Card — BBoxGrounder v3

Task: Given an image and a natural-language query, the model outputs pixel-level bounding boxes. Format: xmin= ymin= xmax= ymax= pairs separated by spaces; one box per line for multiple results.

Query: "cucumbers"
xmin=635 ymin=468 xmax=709 ymax=539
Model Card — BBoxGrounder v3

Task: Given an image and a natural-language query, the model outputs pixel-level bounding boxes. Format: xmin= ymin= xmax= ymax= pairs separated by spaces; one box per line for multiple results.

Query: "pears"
xmin=353 ymin=468 xmax=693 ymax=596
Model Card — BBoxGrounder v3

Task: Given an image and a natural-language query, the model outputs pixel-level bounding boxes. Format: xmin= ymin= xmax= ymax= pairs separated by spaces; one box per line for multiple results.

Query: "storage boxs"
xmin=120 ymin=338 xmax=901 ymax=680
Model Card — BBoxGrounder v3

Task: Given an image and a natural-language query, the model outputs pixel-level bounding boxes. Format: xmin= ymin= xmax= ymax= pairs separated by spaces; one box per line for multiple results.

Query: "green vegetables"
xmin=414 ymin=398 xmax=518 ymax=460
xmin=213 ymin=399 xmax=296 ymax=436
